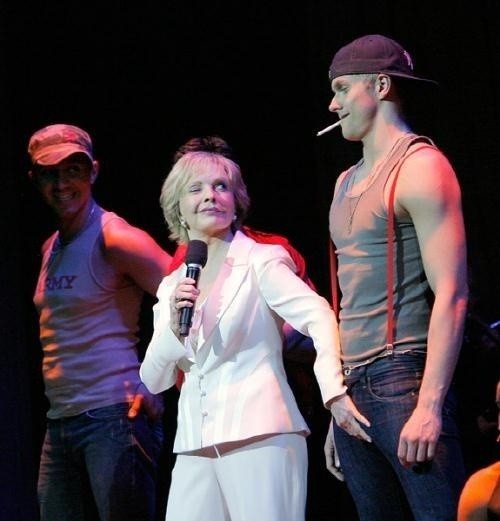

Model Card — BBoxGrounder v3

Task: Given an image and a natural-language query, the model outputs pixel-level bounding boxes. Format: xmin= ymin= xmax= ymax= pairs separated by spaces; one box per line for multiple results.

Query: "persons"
xmin=138 ymin=151 xmax=372 ymax=521
xmin=321 ymin=35 xmax=470 ymax=521
xmin=27 ymin=122 xmax=180 ymax=521
xmin=163 ymin=135 xmax=318 ymax=393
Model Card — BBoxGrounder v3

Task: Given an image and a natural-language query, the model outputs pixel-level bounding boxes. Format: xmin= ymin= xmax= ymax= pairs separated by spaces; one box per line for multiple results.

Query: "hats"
xmin=327 ymin=35 xmax=440 ymax=88
xmin=26 ymin=123 xmax=96 ymax=170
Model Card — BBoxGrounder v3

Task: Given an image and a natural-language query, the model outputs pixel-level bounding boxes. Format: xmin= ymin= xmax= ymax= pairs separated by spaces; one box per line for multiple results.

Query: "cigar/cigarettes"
xmin=316 ymin=119 xmax=343 ymax=138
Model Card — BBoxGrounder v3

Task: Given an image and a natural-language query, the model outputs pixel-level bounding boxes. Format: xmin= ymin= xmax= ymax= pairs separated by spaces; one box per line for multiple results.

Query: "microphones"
xmin=177 ymin=239 xmax=208 ymax=339
